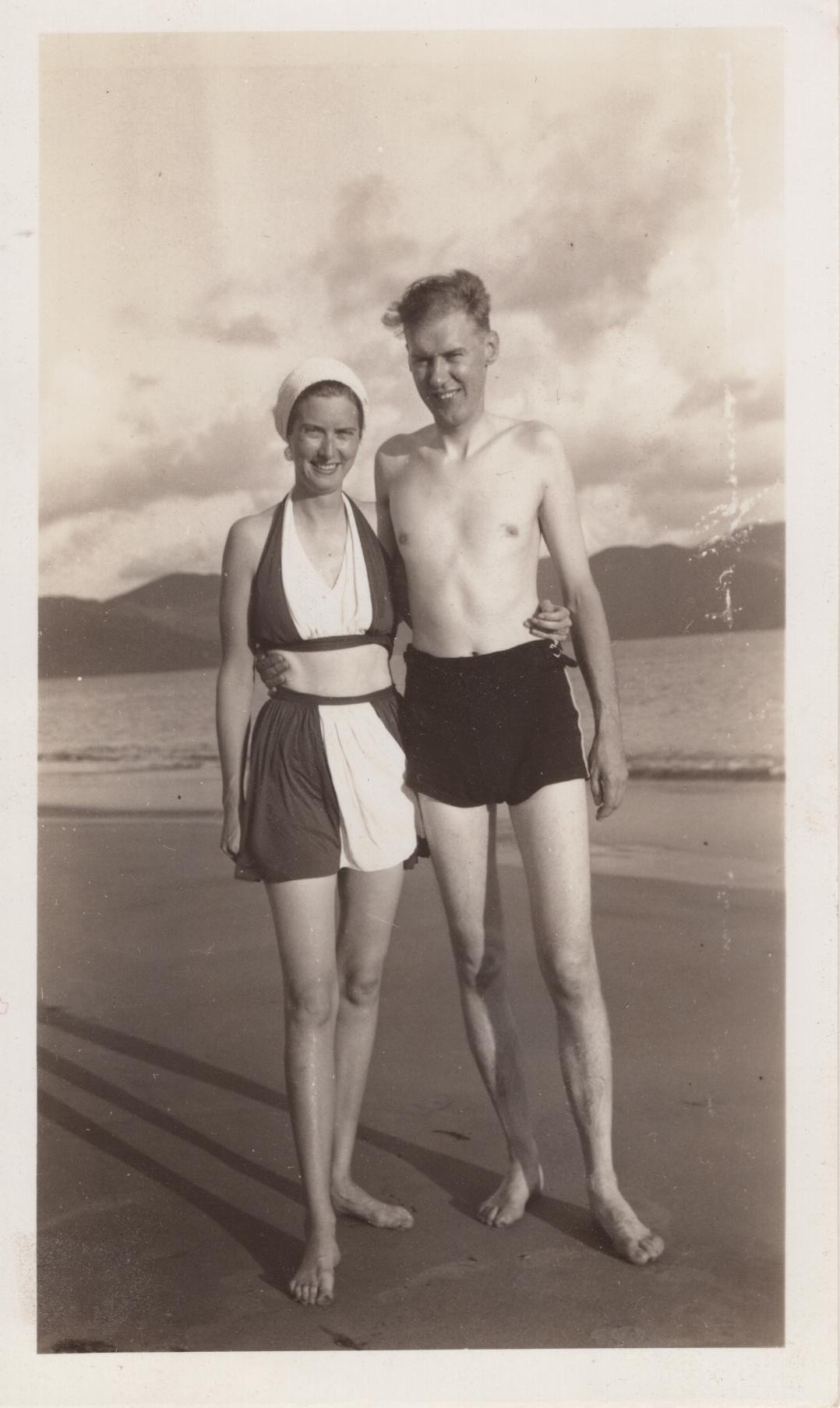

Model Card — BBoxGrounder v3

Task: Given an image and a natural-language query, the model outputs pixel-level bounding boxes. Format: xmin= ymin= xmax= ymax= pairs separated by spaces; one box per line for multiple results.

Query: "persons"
xmin=255 ymin=266 xmax=672 ymax=1273
xmin=207 ymin=357 xmax=578 ymax=1308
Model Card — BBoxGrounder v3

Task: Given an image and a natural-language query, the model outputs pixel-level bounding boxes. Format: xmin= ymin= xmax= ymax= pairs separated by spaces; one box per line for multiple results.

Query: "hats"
xmin=273 ymin=359 xmax=368 ymax=444
